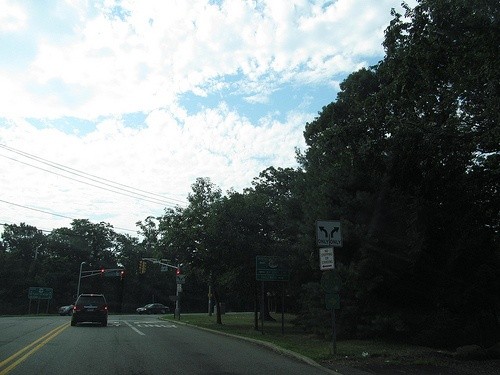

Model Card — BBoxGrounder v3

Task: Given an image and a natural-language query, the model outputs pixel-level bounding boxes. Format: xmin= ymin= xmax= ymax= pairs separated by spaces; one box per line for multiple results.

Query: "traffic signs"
xmin=319 ymin=247 xmax=334 ymax=270
xmin=316 ymin=220 xmax=344 ymax=247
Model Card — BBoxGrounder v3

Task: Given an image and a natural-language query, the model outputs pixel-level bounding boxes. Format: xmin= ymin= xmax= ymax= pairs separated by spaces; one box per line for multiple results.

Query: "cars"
xmin=136 ymin=302 xmax=170 ymax=314
xmin=57 ymin=304 xmax=74 ymax=316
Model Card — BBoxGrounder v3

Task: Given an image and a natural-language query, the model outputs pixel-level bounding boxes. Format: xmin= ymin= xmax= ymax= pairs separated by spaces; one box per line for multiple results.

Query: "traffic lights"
xmin=120 ymin=271 xmax=125 ymax=281
xmin=139 ymin=261 xmax=147 ymax=275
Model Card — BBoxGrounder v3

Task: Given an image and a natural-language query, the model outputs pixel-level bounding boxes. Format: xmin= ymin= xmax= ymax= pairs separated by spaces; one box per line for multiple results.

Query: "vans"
xmin=71 ymin=294 xmax=108 ymax=327
